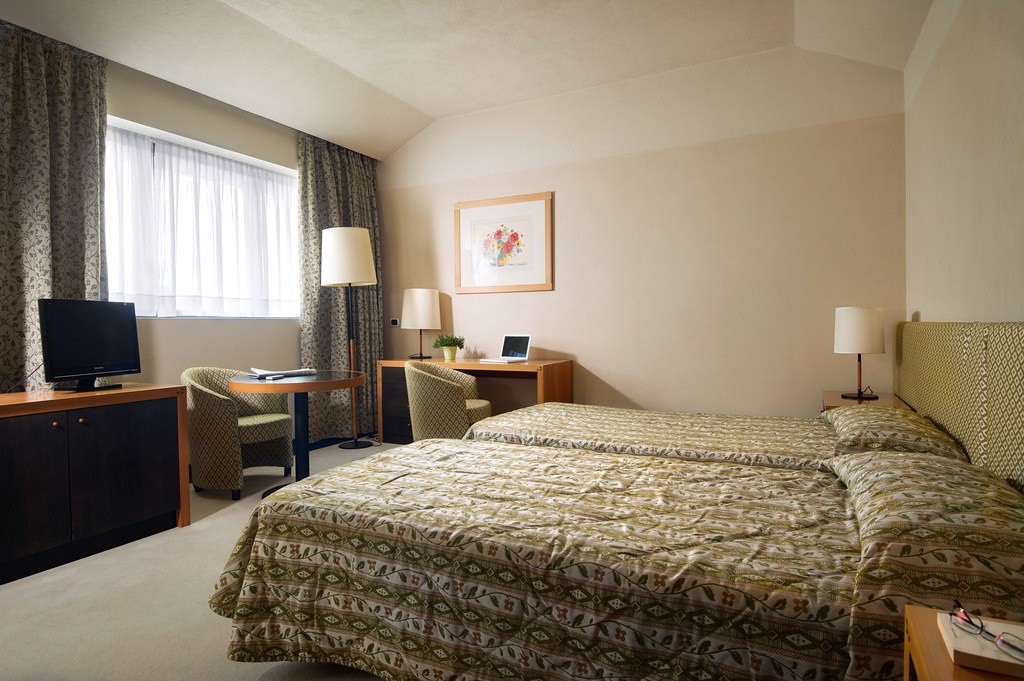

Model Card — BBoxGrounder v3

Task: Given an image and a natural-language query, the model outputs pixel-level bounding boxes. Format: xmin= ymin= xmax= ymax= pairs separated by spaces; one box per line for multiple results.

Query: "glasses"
xmin=949 ymin=598 xmax=1024 ymax=661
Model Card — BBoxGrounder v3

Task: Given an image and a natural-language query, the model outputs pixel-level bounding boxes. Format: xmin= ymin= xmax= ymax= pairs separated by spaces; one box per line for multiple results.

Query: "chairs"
xmin=405 ymin=360 xmax=492 ymax=442
xmin=180 ymin=367 xmax=294 ymax=500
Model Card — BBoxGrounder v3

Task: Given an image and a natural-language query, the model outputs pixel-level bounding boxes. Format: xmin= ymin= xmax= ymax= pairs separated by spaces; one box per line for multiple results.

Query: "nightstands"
xmin=822 ymin=390 xmax=912 ymax=411
xmin=904 ymin=604 xmax=1024 ymax=681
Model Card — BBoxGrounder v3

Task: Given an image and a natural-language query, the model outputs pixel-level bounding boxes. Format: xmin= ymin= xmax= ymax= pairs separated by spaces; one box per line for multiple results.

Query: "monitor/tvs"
xmin=38 ymin=298 xmax=141 ymax=393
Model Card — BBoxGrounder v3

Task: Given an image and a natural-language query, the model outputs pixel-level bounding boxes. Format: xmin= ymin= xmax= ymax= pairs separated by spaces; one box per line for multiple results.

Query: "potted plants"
xmin=432 ymin=333 xmax=465 ymax=360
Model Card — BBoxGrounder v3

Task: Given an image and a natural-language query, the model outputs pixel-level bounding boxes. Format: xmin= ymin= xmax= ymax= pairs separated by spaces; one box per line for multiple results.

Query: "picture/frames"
xmin=454 ymin=191 xmax=554 ymax=295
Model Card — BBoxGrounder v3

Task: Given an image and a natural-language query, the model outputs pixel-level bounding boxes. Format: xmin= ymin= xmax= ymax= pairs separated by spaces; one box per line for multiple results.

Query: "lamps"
xmin=401 ymin=288 xmax=442 ymax=359
xmin=321 ymin=227 xmax=379 ymax=450
xmin=833 ymin=306 xmax=885 ymax=400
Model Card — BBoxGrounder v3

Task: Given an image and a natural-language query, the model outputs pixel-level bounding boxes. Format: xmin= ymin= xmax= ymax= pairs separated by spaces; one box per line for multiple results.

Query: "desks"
xmin=228 ymin=369 xmax=367 ymax=500
xmin=377 ymin=356 xmax=572 ymax=445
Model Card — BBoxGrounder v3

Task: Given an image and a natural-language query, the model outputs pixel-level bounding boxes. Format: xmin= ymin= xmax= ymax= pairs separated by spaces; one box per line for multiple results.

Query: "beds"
xmin=204 ymin=320 xmax=1024 ymax=681
xmin=460 ymin=322 xmax=989 ymax=473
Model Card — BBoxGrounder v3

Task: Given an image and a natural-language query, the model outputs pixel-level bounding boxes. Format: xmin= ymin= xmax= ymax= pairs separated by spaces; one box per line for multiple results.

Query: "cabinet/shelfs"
xmin=0 ymin=382 xmax=188 ymax=586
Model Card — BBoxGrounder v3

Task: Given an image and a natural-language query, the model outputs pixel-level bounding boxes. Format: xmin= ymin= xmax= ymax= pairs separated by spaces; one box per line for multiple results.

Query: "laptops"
xmin=480 ymin=335 xmax=531 ymax=363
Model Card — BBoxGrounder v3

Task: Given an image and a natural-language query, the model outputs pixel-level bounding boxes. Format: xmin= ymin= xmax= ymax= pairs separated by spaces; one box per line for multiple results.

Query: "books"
xmin=936 ymin=612 xmax=1024 ymax=678
